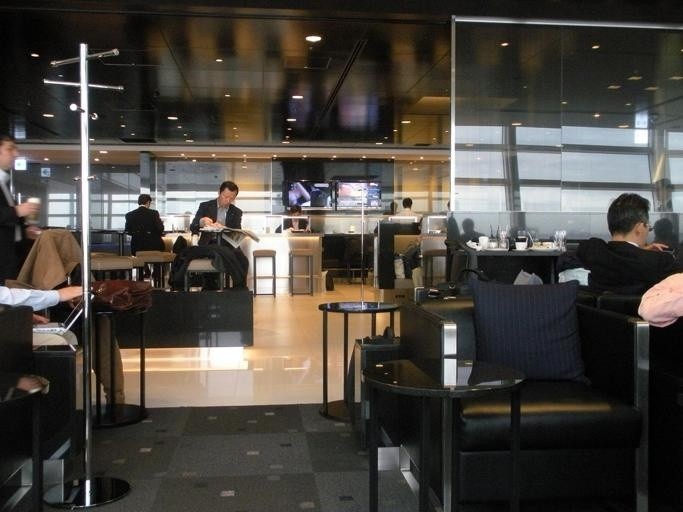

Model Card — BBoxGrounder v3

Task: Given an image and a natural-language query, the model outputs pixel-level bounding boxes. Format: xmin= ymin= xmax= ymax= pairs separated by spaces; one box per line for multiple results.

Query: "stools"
xmin=89 ymin=249 xmax=176 ymax=292
xmin=252 ymin=248 xmax=314 ymax=298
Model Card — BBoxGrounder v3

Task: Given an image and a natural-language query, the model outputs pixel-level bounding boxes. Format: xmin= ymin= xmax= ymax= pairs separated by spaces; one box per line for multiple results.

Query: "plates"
xmin=483 ymin=247 xmax=559 ymax=252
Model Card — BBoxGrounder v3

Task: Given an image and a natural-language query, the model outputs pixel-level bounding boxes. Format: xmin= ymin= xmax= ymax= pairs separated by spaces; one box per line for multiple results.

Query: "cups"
xmin=24 ymin=197 xmax=42 ymax=224
xmin=476 ymin=229 xmax=568 ymax=248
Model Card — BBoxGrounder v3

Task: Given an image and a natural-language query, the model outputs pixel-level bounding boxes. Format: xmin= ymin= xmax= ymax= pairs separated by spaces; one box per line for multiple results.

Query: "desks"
xmin=449 ymin=248 xmax=563 ymax=286
xmin=317 ymin=300 xmax=528 ymax=511
xmin=72 ymin=296 xmax=153 ymax=430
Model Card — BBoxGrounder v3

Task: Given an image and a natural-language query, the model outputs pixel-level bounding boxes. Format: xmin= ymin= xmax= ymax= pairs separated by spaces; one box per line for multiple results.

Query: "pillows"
xmin=468 ymin=279 xmax=591 ymax=387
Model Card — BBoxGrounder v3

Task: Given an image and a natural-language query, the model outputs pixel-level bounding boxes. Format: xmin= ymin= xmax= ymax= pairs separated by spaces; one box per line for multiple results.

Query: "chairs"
xmin=398 ymin=297 xmax=649 ymax=511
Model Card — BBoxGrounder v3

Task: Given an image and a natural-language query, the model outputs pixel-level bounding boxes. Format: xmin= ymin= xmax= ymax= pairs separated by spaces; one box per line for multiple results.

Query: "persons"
xmin=274 ymin=205 xmax=315 ymax=235
xmin=166 ymin=179 xmax=249 ymax=292
xmin=654 ymin=177 xmax=675 ymax=208
xmin=122 ymin=194 xmax=166 ymax=289
xmin=575 ymin=193 xmax=683 ymax=281
xmin=0 ymin=280 xmax=93 ymax=325
xmin=396 ymin=198 xmax=423 ymax=219
xmin=1 ymin=130 xmax=42 ymax=326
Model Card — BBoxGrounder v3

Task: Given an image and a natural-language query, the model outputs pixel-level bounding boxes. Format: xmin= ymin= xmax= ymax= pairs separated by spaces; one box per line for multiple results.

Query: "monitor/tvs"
xmin=334 ymin=180 xmax=383 ymax=211
xmin=282 ymin=178 xmax=333 ymax=210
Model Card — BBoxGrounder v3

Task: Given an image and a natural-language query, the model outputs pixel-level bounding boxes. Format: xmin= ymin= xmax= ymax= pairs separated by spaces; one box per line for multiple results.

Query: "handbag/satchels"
xmin=394 ymin=242 xmax=418 ymax=278
xmin=71 ymin=280 xmax=153 ymax=311
